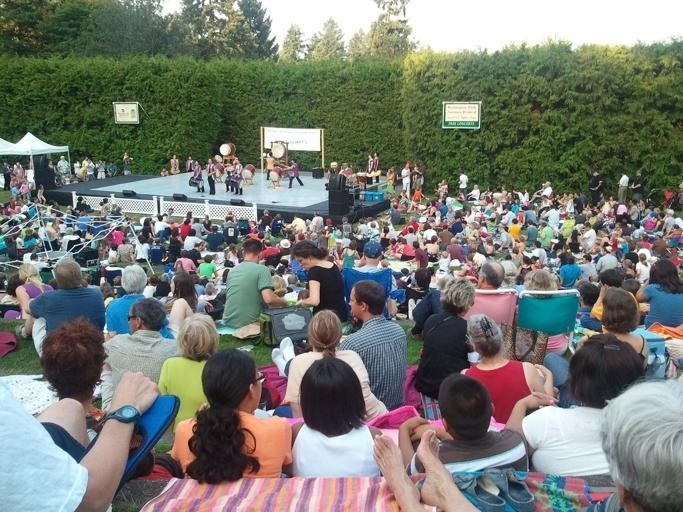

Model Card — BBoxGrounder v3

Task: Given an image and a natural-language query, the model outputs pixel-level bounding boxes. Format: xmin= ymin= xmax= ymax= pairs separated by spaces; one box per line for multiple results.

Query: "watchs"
xmin=104 ymin=405 xmax=142 ymax=423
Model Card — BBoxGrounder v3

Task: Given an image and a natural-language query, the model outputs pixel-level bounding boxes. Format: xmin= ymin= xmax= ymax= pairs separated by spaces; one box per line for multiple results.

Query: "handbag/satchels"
xmin=498 ymin=321 xmax=548 ymax=365
xmin=258 ymin=387 xmax=281 ymax=410
xmin=423 ymin=315 xmax=457 ymax=342
xmin=666 ymin=349 xmax=679 ymax=378
xmin=260 ymin=308 xmax=313 ymax=347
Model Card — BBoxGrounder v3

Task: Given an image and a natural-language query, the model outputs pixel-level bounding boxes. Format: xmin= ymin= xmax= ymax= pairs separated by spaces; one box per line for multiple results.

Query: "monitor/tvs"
xmin=349 ymin=188 xmax=360 ymax=203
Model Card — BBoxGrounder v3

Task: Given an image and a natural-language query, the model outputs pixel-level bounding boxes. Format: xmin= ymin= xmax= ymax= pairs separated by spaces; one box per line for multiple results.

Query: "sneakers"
xmin=484 ymin=465 xmax=535 ymax=512
xmin=408 ymin=299 xmax=416 ymax=321
xmin=451 ymin=469 xmax=506 ymax=512
xmin=418 ymin=298 xmax=423 ymax=304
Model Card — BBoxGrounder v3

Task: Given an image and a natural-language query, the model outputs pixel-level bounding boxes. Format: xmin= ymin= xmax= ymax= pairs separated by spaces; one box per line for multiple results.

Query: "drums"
xmin=212 ymin=155 xmax=222 ymax=165
xmin=215 ymin=163 xmax=224 ymax=177
xmin=357 ymin=173 xmax=367 ymax=184
xmin=189 ymin=177 xmax=204 ymax=188
xmin=242 ymin=164 xmax=256 ymax=178
xmin=230 ymin=176 xmax=244 ymax=189
xmin=272 ymin=143 xmax=286 ymax=159
xmin=220 ymin=143 xmax=236 ymax=155
xmin=270 ymin=167 xmax=282 ymax=181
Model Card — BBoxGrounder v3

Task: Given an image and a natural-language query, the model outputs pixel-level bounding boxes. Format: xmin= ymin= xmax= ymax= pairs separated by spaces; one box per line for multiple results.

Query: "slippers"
xmin=14 ymin=324 xmax=31 ymax=339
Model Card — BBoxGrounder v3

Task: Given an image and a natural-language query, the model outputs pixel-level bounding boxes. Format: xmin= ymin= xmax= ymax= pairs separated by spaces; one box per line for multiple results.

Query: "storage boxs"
xmin=359 ymin=191 xmax=366 ymax=201
xmin=373 ymin=192 xmax=384 ymax=200
xmin=364 ymin=192 xmax=373 ymax=200
xmin=252 ymin=305 xmax=314 ymax=347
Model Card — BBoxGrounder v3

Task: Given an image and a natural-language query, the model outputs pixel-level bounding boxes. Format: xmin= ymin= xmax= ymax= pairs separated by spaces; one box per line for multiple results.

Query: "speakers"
xmin=312 ymin=167 xmax=324 ymax=178
xmin=376 ymin=176 xmax=379 ymax=183
xmin=328 ymin=174 xmax=346 ymax=191
xmin=328 ymin=194 xmax=348 ymax=213
xmin=367 ymin=177 xmax=372 ymax=183
xmin=35 ymin=168 xmax=55 ymax=188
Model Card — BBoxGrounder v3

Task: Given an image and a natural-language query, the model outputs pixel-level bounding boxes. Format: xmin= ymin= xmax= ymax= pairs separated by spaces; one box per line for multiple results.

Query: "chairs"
xmin=342 ymin=266 xmax=392 ymax=323
xmin=516 ymin=287 xmax=580 ymax=355
xmin=455 ymin=288 xmax=517 ymax=332
xmin=76 ymin=393 xmax=181 ymax=493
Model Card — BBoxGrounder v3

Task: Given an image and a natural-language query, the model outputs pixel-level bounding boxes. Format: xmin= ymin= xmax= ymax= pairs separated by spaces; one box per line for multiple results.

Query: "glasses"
xmin=126 ymin=314 xmax=138 ymax=324
xmin=250 ymin=371 xmax=266 ymax=385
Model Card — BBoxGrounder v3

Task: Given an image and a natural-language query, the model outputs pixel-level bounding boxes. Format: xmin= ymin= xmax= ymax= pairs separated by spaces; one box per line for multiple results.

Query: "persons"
xmin=575 ymin=287 xmax=647 ymax=371
xmin=351 ymin=240 xmax=396 ymax=316
xmin=460 ymin=314 xmax=554 ymax=424
xmin=413 ymin=279 xmax=476 ymax=400
xmin=271 ymin=279 xmax=408 ymax=411
xmin=295 ymin=240 xmax=347 ymax=323
xmin=17 ymin=370 xmax=161 ymax=512
xmin=170 ymin=155 xmax=179 ymax=174
xmin=108 ymin=208 xmax=322 ymax=271
xmin=576 ymin=283 xmax=599 ymax=329
xmin=0 ymin=198 xmax=112 ymax=265
xmin=590 ymin=269 xmax=621 ymax=321
xmin=159 ymin=168 xmax=167 ymax=176
xmin=185 ymin=155 xmax=193 ymax=172
xmin=457 ymin=170 xmax=468 ymax=199
xmin=629 ymin=171 xmax=646 ymax=202
xmin=588 ymin=169 xmax=601 ymax=206
xmin=291 ymin=358 xmax=385 ymax=478
xmin=167 ymin=272 xmax=199 ymax=340
xmin=266 ymin=152 xmax=275 ymax=180
xmin=221 ymin=238 xmax=289 ymax=329
xmin=3 ymin=263 xmax=54 ymax=341
xmin=412 ymin=162 xmax=423 ymax=192
xmin=620 ymin=278 xmax=641 ymax=296
xmin=157 ymin=313 xmax=219 ymax=433
xmin=386 ymin=167 xmax=395 ymax=194
xmin=324 ymin=181 xmax=683 ymax=289
xmin=99 ymin=297 xmax=183 ymax=411
xmin=406 ymin=261 xmax=505 ymax=342
xmin=372 ymin=429 xmax=482 ymax=511
xmin=205 ymin=159 xmax=217 ymax=195
xmin=398 ymin=372 xmax=529 ymax=477
xmin=19 ymin=260 xmax=104 ymax=360
xmin=192 ymin=160 xmax=205 ymax=193
xmin=105 ymin=264 xmax=174 ymax=340
xmin=281 ymin=159 xmax=303 ymax=188
xmin=372 ymin=152 xmax=380 ymax=183
xmin=223 ymin=160 xmax=242 ymax=195
xmin=272 ymin=309 xmax=389 ymax=426
xmin=1 ymin=157 xmax=118 ymax=199
xmin=367 ymin=155 xmax=373 ymax=185
xmin=400 ymin=161 xmax=414 ymax=201
xmin=615 ymin=171 xmax=629 ymax=205
xmin=114 ymin=154 xmax=130 ymax=175
xmin=595 ymin=378 xmax=682 ymax=512
xmin=39 ymin=318 xmax=109 ymax=433
xmin=170 ymin=347 xmax=293 ymax=478
xmin=504 ymin=333 xmax=645 ymax=476
xmin=635 ymin=259 xmax=682 ymax=329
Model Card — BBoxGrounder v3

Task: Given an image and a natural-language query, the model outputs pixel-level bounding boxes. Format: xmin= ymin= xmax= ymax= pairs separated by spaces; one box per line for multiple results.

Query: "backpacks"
xmin=259 ymin=304 xmax=314 ymax=345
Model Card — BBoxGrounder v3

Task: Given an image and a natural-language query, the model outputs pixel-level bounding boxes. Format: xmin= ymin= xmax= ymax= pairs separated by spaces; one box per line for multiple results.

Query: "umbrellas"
xmin=1 ymin=131 xmax=70 ymax=188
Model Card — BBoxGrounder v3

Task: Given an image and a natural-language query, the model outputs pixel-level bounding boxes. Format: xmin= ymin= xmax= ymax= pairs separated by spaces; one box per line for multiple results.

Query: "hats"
xmin=364 ymin=241 xmax=381 ymax=258
xmin=279 ymin=238 xmax=291 ymax=249
xmin=467 ymin=315 xmax=499 ymax=341
